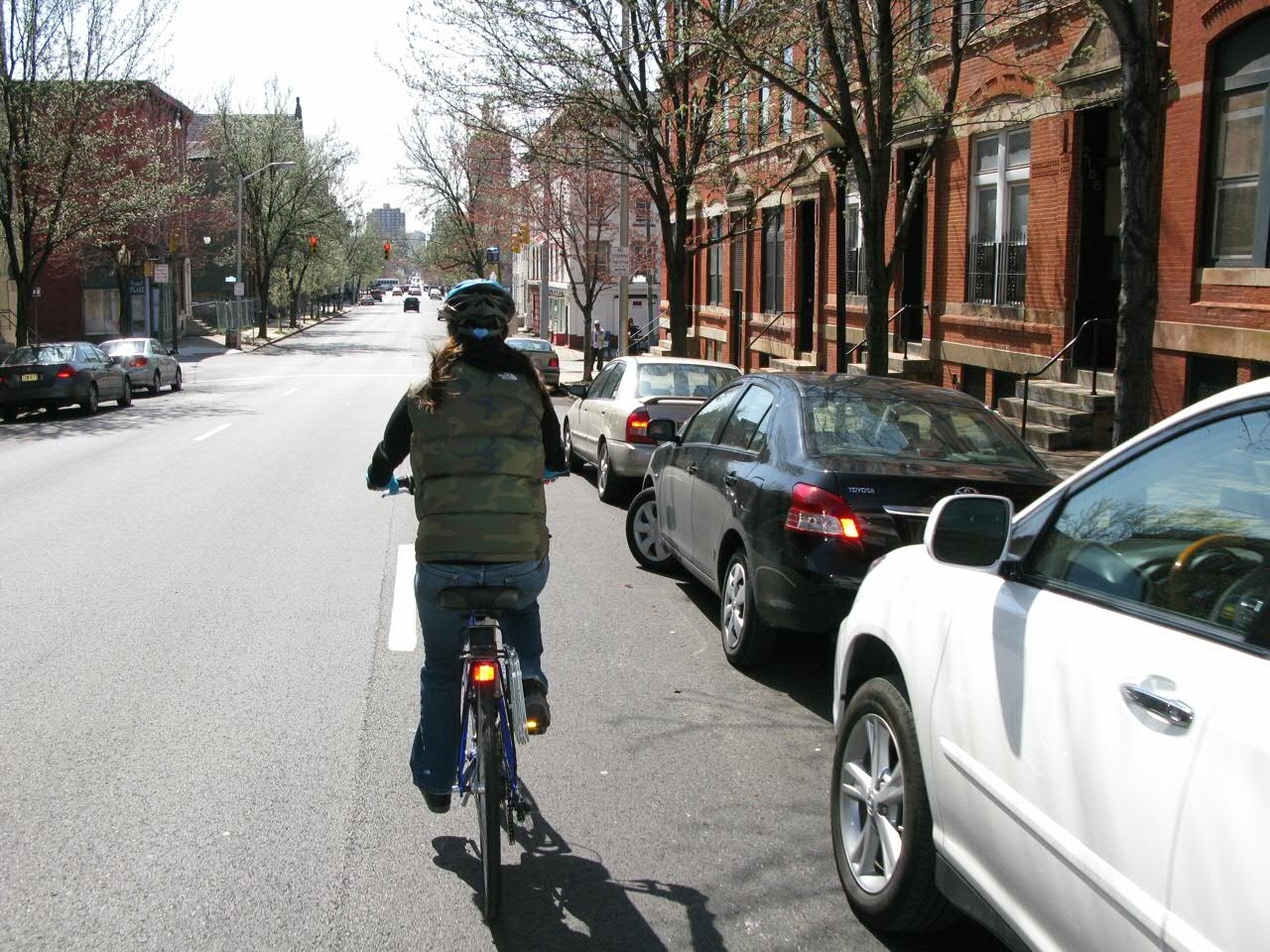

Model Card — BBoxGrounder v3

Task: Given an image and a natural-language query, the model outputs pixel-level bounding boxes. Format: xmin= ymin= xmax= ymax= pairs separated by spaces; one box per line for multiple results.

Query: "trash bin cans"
xmin=225 ymin=328 xmax=238 ymax=350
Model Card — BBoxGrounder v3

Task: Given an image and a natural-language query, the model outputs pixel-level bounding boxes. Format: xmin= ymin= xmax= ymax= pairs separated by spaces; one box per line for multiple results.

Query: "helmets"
xmin=436 ymin=279 xmax=517 ymax=337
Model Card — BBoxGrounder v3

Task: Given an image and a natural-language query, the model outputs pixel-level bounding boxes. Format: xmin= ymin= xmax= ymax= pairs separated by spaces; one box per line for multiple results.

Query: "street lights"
xmin=236 ymin=161 xmax=298 ymax=349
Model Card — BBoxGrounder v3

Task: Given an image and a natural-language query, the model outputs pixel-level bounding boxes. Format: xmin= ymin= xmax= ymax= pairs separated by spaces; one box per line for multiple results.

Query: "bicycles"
xmin=380 ymin=469 xmax=572 ymax=919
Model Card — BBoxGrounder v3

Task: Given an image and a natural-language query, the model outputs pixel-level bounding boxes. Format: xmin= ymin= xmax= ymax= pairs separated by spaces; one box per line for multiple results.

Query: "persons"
xmin=590 ymin=320 xmax=605 ymax=372
xmin=367 ymin=280 xmax=566 ymax=814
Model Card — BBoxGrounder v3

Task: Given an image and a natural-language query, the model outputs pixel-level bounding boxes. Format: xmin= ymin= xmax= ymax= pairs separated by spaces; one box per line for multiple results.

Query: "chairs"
xmin=885 ymin=421 xmax=920 ymax=449
xmin=692 ymin=385 xmax=715 ymax=396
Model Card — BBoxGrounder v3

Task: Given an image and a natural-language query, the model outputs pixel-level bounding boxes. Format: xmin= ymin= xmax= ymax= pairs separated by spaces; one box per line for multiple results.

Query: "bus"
xmin=374 ymin=278 xmax=398 ymax=290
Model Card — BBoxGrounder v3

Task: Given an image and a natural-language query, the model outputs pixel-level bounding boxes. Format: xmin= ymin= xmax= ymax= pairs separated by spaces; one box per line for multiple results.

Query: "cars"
xmin=625 ymin=372 xmax=1067 ymax=669
xmin=505 ymin=337 xmax=561 ymax=386
xmin=359 ymin=282 xmax=442 ymax=305
xmin=563 ymin=356 xmax=746 ymax=503
xmin=403 ymin=297 xmax=422 ymax=312
xmin=828 ymin=375 xmax=1270 ymax=952
xmin=436 ymin=305 xmax=445 ymax=321
xmin=98 ymin=338 xmax=183 ymax=395
xmin=0 ymin=341 xmax=132 ymax=423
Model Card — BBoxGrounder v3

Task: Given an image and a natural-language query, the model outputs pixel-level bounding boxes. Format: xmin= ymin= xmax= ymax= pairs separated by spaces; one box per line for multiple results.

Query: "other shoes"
xmin=526 ymin=686 xmax=551 ymax=727
xmin=418 ymin=785 xmax=451 ymax=813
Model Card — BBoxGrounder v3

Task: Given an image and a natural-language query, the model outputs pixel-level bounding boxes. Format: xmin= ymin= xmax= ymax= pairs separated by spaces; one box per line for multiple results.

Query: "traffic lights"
xmin=311 ymin=237 xmax=317 ymax=253
xmin=520 ymin=223 xmax=530 ymax=245
xmin=510 ymin=234 xmax=521 ymax=254
xmin=384 ymin=244 xmax=391 ymax=259
